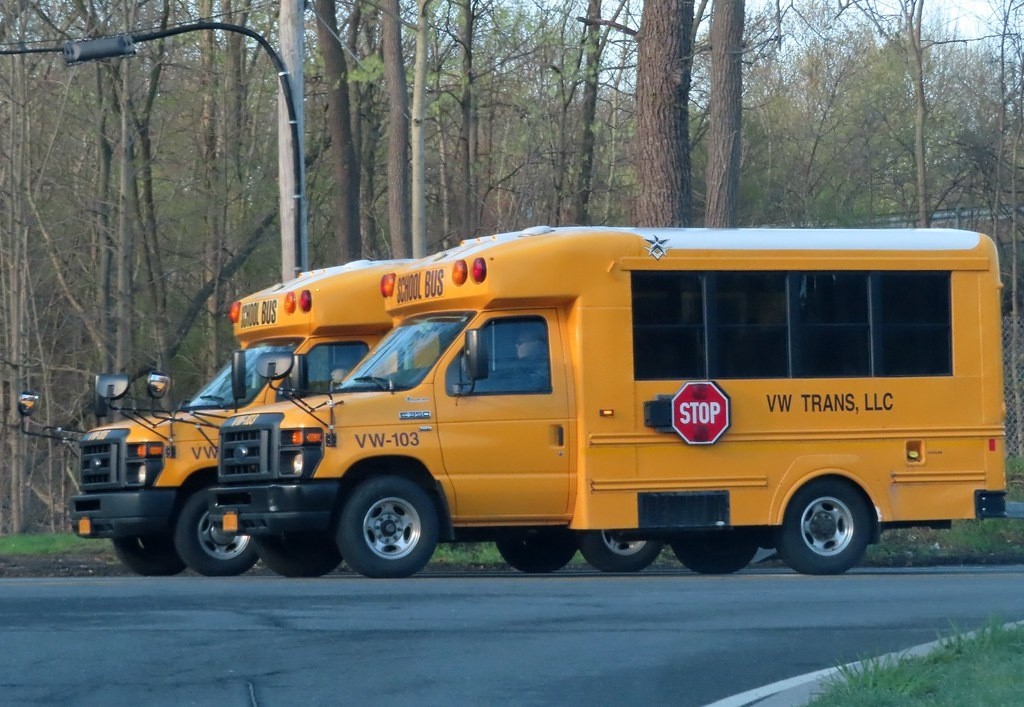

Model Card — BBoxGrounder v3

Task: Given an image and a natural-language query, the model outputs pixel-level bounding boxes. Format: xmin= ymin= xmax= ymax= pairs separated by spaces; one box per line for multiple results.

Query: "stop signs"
xmin=670 ymin=381 xmax=730 ymax=446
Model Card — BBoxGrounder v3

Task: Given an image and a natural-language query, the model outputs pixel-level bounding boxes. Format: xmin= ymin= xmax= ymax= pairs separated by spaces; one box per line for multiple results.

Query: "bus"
xmin=147 ymin=219 xmax=1010 ymax=580
xmin=14 ymin=258 xmax=425 ymax=578
